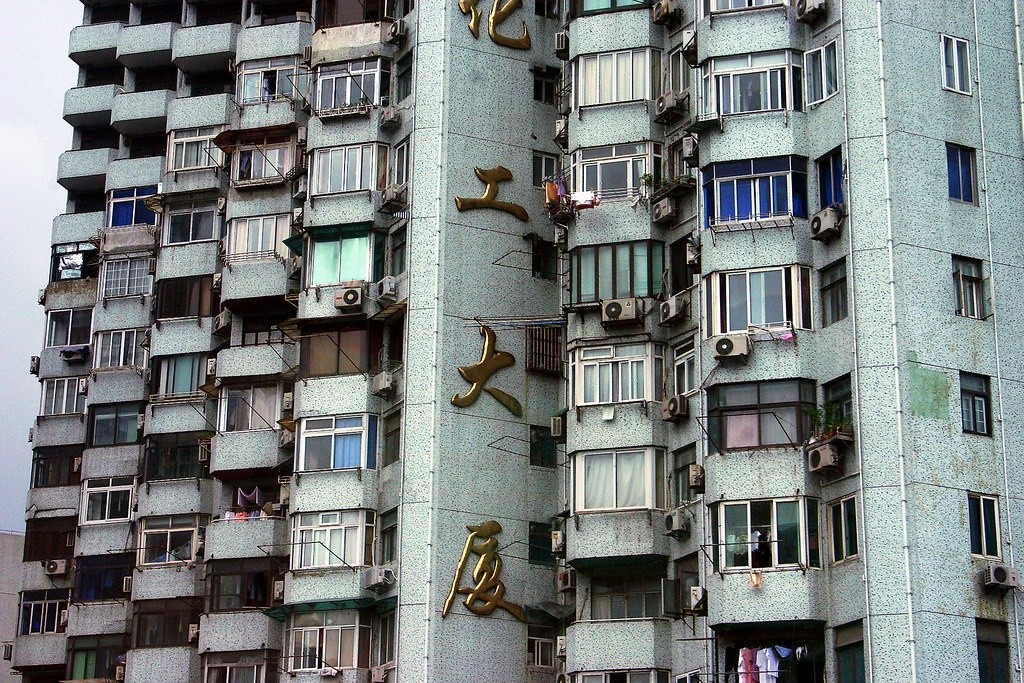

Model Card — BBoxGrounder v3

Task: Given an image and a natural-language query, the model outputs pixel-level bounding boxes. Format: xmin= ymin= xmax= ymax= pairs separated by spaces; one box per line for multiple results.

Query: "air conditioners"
xmin=320 ymin=17 xmax=408 ymax=683
xmin=3 ymin=644 xmax=12 ymax=661
xmin=809 ymin=208 xmax=838 ymax=238
xmin=38 ymin=288 xmax=44 ymax=302
xmin=30 ymin=356 xmax=40 ymax=376
xmin=551 ymin=0 xmax=707 ymax=683
xmin=984 ymin=565 xmax=1018 ymax=589
xmin=713 ymin=336 xmax=747 ymax=358
xmin=116 ymin=257 xmax=156 ymax=682
xmin=808 ymin=444 xmax=838 ymax=472
xmin=44 ymin=377 xmax=88 ymax=626
xmin=189 ymin=196 xmax=231 ymax=643
xmin=795 ymin=0 xmax=825 ymax=22
xmin=273 ymin=43 xmax=313 ymax=602
